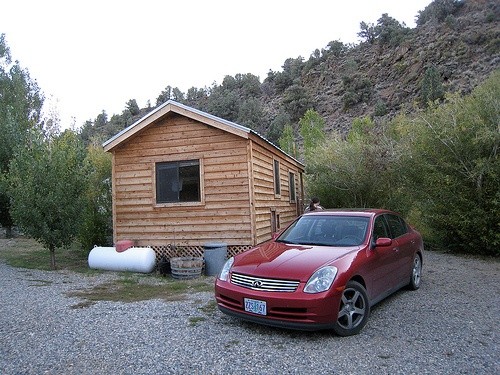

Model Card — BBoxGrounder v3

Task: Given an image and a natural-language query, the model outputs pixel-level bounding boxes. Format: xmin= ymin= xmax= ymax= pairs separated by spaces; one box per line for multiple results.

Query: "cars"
xmin=215 ymin=208 xmax=425 ymax=337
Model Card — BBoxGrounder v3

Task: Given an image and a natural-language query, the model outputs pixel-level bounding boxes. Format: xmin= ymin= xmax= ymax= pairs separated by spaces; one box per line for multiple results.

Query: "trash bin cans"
xmin=201 ymin=243 xmax=228 ymax=277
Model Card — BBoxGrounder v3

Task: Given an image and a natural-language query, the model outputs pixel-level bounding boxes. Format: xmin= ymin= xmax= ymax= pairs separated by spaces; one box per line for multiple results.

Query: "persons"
xmin=304 ymin=196 xmax=325 ymax=213
